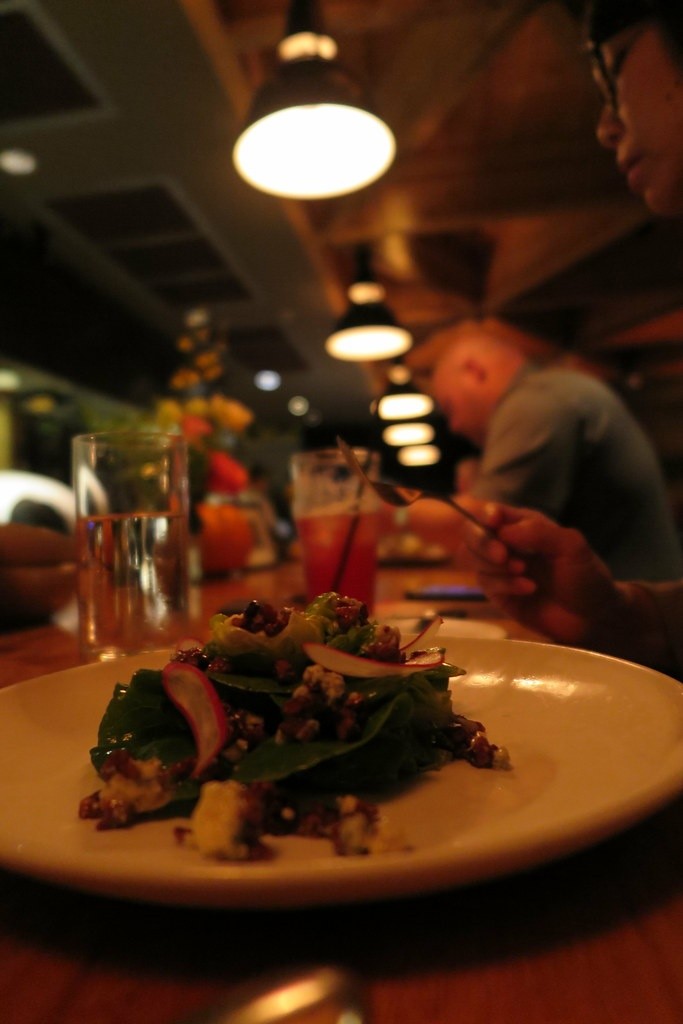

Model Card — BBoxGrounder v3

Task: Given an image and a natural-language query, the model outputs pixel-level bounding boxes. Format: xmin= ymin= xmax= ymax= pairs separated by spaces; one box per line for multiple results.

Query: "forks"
xmin=338 ymin=437 xmax=509 ymax=546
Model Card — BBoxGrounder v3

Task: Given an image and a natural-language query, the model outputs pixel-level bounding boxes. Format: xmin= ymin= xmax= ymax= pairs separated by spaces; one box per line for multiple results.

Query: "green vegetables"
xmin=90 ymin=591 xmax=465 ymax=787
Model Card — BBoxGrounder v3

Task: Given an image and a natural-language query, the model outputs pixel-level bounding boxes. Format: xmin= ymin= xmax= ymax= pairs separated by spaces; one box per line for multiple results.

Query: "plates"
xmin=0 ymin=636 xmax=683 ymax=902
xmin=376 ymin=617 xmax=507 ymax=643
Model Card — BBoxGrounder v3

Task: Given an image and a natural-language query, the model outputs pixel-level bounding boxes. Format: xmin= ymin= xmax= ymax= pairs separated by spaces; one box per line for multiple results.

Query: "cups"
xmin=287 ymin=449 xmax=382 ymax=621
xmin=198 ymin=494 xmax=271 ymax=575
xmin=70 ymin=433 xmax=191 ymax=663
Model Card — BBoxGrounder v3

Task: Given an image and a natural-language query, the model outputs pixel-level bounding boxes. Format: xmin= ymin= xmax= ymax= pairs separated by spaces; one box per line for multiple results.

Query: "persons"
xmin=452 ymin=1 xmax=683 ymax=682
xmin=1 ymin=518 xmax=78 ymax=634
xmin=382 ymin=321 xmax=683 ymax=586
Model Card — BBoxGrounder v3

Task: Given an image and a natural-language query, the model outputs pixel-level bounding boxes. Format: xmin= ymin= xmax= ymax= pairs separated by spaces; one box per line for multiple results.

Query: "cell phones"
xmin=404 ymin=588 xmax=486 ymax=601
